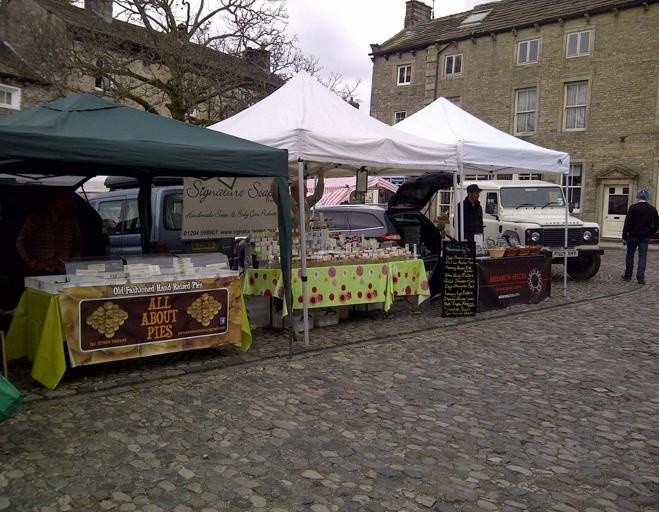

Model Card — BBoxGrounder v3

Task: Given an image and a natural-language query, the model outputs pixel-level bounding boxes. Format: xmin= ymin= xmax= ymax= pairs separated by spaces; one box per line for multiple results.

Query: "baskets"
xmin=485 ymin=247 xmax=544 ymax=257
xmin=284 ymin=312 xmax=314 ymax=332
xmin=314 ymin=309 xmax=339 ymax=328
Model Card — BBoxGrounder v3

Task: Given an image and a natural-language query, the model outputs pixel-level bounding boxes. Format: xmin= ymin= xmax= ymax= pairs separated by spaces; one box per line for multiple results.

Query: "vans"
xmin=87 ymin=184 xmax=237 ymax=269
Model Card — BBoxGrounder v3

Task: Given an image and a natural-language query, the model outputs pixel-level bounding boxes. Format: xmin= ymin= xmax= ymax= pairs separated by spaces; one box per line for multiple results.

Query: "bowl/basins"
xmin=486 ymin=246 xmax=543 ymax=257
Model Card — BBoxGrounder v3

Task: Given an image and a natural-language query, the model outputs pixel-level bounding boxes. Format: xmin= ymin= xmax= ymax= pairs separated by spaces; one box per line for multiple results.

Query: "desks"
xmin=247 ymin=256 xmax=425 ymax=349
xmin=440 ymin=250 xmax=553 ymax=312
xmin=24 ymin=275 xmax=244 ymax=391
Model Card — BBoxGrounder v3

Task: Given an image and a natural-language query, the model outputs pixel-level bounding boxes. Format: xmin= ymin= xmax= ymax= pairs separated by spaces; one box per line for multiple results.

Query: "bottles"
xmin=420 ymin=243 xmax=426 ymax=258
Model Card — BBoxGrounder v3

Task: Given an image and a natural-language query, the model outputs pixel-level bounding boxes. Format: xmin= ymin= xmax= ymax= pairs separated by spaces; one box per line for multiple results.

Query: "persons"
xmin=452 ymin=185 xmax=484 ymax=242
xmin=16 ymin=190 xmax=85 ymax=277
xmin=621 ymin=190 xmax=659 ymax=286
xmin=270 ymin=166 xmax=325 ymax=314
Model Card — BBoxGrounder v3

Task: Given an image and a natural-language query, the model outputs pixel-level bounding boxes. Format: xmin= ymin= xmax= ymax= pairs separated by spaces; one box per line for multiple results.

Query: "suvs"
xmin=456 ymin=179 xmax=604 ymax=280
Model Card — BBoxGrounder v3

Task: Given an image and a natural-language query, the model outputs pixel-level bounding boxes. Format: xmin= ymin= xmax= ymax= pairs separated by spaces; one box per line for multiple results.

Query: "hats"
xmin=467 ymin=184 xmax=482 ymax=192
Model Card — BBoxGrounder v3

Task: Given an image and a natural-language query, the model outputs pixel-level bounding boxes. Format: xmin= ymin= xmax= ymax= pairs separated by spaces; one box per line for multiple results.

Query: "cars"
xmin=240 ymin=204 xmax=402 ymax=268
